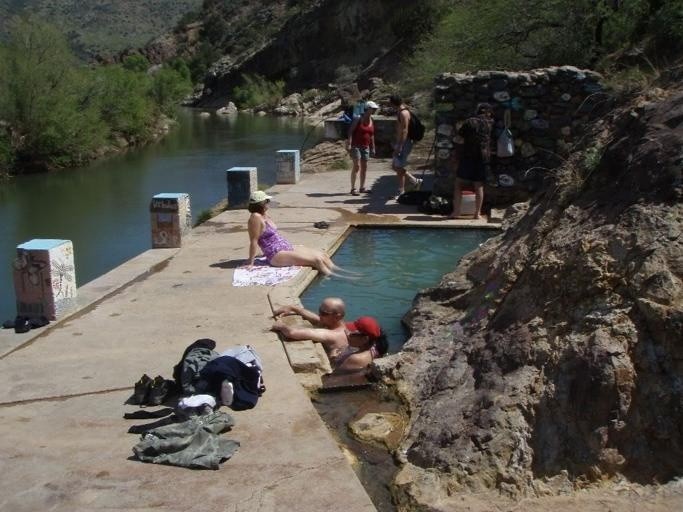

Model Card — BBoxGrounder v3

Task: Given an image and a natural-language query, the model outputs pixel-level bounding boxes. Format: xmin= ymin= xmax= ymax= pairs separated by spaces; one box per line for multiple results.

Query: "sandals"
xmin=351 ymin=187 xmax=371 ymax=196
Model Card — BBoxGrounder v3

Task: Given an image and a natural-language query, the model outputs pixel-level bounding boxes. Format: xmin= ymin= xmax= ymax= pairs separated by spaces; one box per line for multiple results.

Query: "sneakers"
xmin=151 ymin=375 xmax=176 ymax=405
xmin=393 ymin=191 xmax=407 ymax=201
xmin=135 ymin=374 xmax=154 ymax=403
xmin=414 ymin=178 xmax=425 ymax=191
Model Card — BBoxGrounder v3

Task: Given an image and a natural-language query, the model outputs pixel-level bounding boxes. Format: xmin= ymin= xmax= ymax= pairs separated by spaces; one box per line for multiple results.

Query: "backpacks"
xmin=399 ymin=108 xmax=425 ymax=141
xmin=497 ymin=127 xmax=514 ymax=158
xmin=471 ymin=118 xmax=498 ymax=170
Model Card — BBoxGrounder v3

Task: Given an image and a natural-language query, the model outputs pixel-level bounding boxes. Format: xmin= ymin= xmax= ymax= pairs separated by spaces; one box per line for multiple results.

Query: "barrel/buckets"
xmin=460 ymin=191 xmax=476 ymax=214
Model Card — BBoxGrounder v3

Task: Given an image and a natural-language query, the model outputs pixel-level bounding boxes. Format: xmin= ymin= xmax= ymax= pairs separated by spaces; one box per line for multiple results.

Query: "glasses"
xmin=262 ymin=199 xmax=270 ymax=204
xmin=349 ymin=332 xmax=365 ymax=337
xmin=319 ymin=310 xmax=336 ymax=315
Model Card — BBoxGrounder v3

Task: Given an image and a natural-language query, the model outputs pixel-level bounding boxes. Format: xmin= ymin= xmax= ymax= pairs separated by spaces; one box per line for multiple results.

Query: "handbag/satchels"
xmin=397 ymin=191 xmax=432 ymax=205
xmin=418 ymin=190 xmax=453 ymax=215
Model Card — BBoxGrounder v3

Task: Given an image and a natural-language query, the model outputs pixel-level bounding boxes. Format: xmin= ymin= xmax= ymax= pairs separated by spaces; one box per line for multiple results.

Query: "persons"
xmin=237 ymin=189 xmax=366 ymax=282
xmin=269 ymin=295 xmax=350 ymax=372
xmin=344 ymin=101 xmax=379 ymax=196
xmin=331 ymin=315 xmax=389 ymax=374
xmin=448 ymin=101 xmax=499 ymax=220
xmin=389 ymin=93 xmax=425 ymax=200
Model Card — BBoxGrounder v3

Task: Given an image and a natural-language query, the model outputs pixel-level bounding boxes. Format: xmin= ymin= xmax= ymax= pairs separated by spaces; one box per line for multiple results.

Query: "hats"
xmin=249 ymin=190 xmax=273 ymax=204
xmin=345 ymin=316 xmax=381 ymax=338
xmin=477 ymin=103 xmax=495 ymax=117
xmin=365 ymin=100 xmax=380 ymax=109
